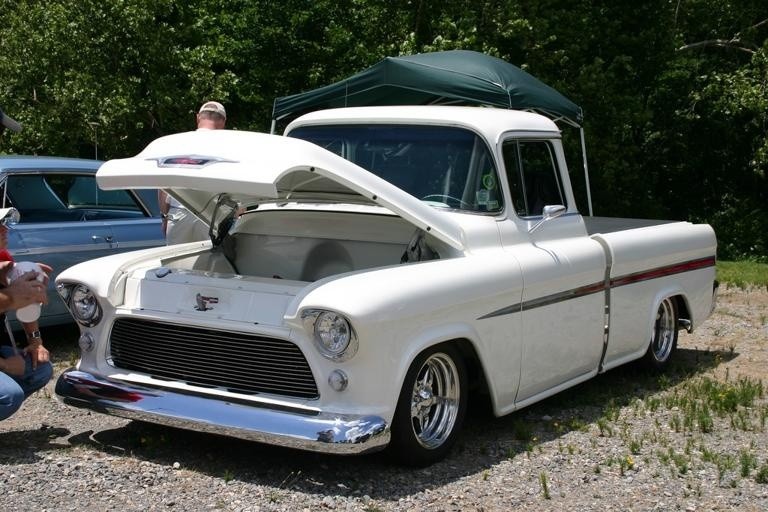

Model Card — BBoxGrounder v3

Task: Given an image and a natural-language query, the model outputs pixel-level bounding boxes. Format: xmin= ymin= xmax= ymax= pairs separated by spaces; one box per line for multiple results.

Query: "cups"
xmin=5 ymin=261 xmax=44 ymax=323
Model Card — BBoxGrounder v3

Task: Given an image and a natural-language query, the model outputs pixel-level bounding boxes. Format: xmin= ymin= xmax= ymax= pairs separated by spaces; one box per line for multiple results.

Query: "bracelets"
xmin=26 ymin=330 xmax=41 ymax=339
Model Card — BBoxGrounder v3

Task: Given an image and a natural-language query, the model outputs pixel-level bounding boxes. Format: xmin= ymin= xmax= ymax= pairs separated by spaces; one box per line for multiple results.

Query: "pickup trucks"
xmin=54 ymin=106 xmax=719 ymax=470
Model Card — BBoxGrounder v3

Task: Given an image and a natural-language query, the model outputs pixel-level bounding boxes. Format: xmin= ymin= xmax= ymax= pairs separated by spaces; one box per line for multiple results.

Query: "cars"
xmin=0 ymin=155 xmax=169 ymax=333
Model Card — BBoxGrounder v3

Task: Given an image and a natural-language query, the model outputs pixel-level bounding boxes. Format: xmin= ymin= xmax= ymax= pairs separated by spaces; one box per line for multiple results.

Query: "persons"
xmin=0 ymin=205 xmax=55 ymax=422
xmin=1 ymin=259 xmax=54 ymax=317
xmin=157 ymin=101 xmax=248 ymax=247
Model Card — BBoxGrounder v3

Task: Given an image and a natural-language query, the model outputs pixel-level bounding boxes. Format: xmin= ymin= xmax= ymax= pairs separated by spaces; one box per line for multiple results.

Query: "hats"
xmin=0 ymin=206 xmax=20 ymax=229
xmin=200 ymin=101 xmax=227 ymax=119
